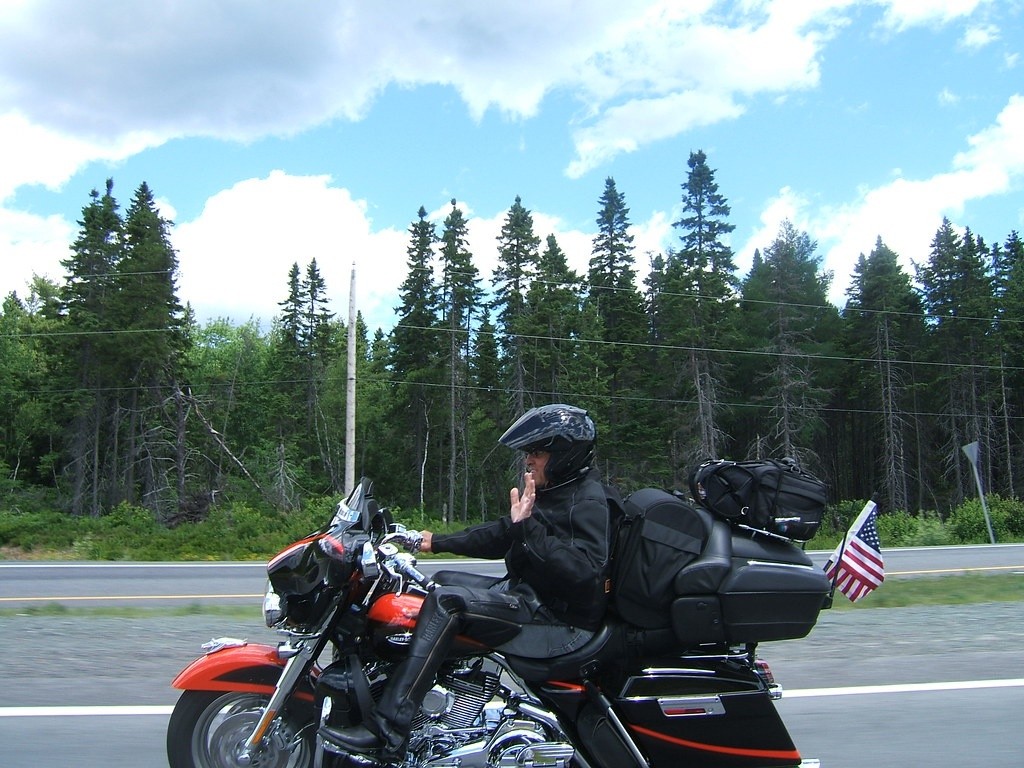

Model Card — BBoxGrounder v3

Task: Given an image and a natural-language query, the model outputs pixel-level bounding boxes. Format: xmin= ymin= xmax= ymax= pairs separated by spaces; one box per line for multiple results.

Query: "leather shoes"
xmin=317 ymin=724 xmax=410 ymax=763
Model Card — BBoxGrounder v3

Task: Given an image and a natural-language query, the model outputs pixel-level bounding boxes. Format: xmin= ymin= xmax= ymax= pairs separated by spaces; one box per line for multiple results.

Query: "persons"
xmin=316 ymin=404 xmax=609 ymax=762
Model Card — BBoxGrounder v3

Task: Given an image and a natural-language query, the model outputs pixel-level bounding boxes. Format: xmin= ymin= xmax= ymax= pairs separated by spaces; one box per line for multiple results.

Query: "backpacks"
xmin=689 ymin=454 xmax=829 ymax=542
xmin=603 ymin=478 xmax=708 ymax=632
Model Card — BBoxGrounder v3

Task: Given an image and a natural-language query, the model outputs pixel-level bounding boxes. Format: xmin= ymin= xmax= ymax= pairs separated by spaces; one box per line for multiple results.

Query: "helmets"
xmin=497 ymin=404 xmax=597 ymax=492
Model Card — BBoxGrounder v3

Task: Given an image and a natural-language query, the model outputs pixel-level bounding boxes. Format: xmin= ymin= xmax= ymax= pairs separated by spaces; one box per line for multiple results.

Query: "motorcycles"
xmin=165 ymin=476 xmax=837 ymax=768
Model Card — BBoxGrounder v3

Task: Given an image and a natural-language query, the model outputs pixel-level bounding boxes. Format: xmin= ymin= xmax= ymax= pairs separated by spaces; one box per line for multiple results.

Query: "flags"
xmin=823 ymin=500 xmax=884 ymax=603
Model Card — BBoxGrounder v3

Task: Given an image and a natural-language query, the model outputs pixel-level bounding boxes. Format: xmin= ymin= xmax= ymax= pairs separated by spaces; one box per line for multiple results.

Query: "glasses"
xmin=523 ymin=446 xmax=544 ymax=459
